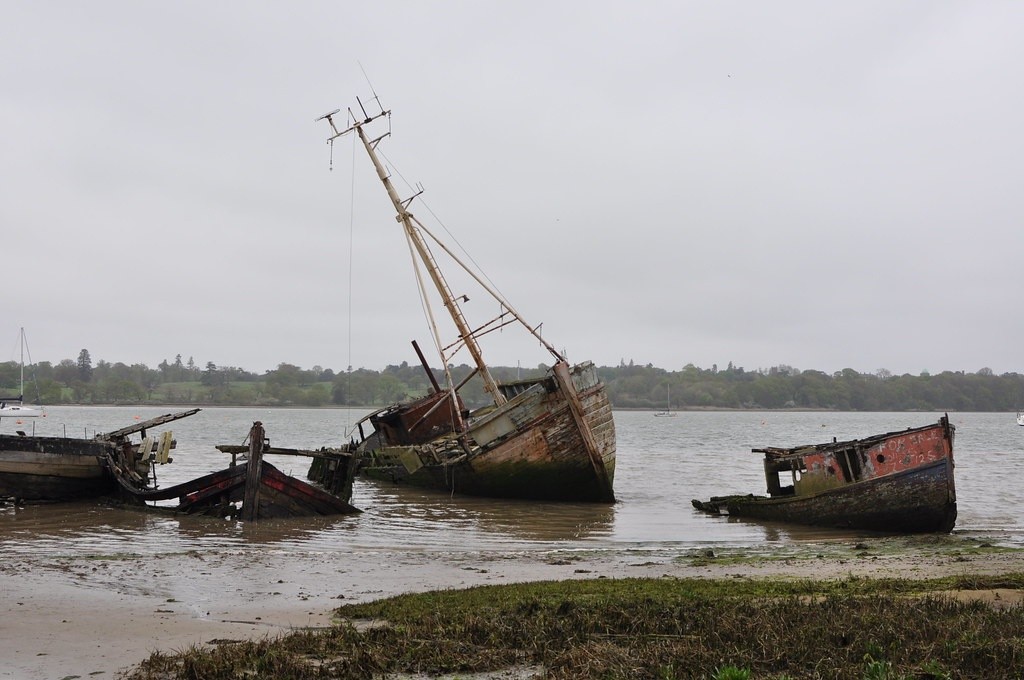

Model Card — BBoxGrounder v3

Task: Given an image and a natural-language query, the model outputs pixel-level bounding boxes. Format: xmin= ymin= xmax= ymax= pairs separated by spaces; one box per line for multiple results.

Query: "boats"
xmin=691 ymin=412 xmax=959 ymax=537
xmin=174 ymin=419 xmax=364 ymax=520
xmin=0 ymin=407 xmax=204 ymax=506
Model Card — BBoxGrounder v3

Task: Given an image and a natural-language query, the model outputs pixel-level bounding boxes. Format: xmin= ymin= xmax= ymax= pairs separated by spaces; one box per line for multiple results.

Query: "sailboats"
xmin=313 ymin=59 xmax=620 ymax=507
xmin=0 ymin=327 xmax=45 ymax=417
